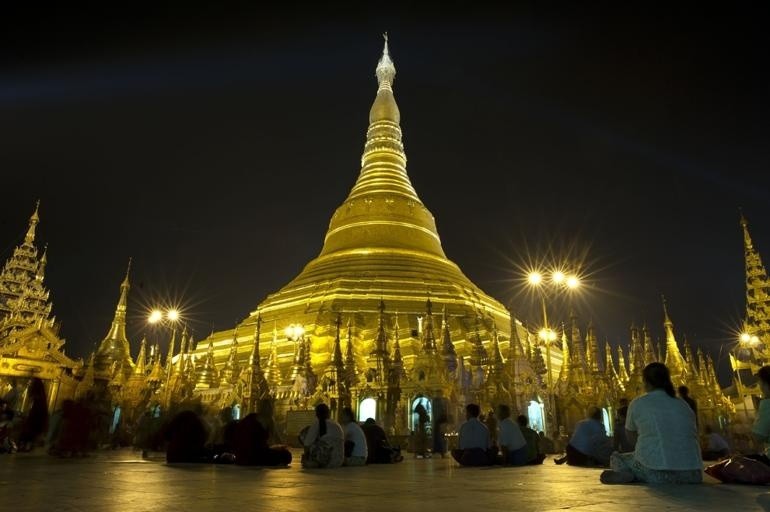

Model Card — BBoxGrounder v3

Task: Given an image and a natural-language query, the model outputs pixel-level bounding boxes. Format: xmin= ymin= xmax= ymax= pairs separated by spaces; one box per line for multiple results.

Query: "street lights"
xmin=284 ymin=323 xmax=306 ymax=384
xmin=147 ymin=308 xmax=180 ymax=414
xmin=731 ymin=331 xmax=760 ymax=446
xmin=524 ymin=269 xmax=582 ymax=391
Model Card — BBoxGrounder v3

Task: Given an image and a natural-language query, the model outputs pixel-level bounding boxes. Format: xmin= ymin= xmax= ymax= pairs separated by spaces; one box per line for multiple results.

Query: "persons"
xmin=724 ymin=363 xmax=769 ymax=486
xmin=598 ymin=361 xmax=705 ymax=486
xmin=2 ymin=375 xmax=753 ymax=470
